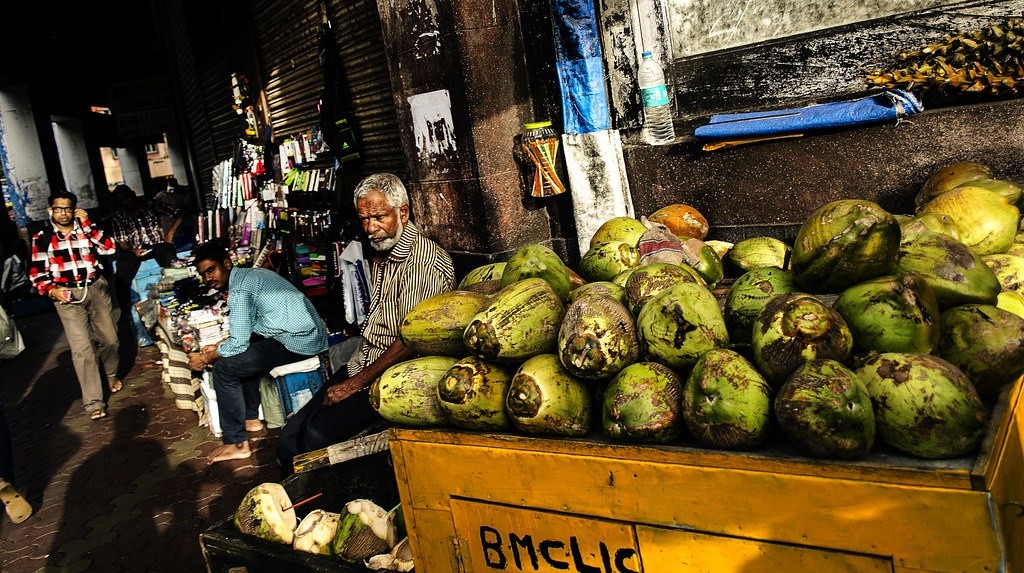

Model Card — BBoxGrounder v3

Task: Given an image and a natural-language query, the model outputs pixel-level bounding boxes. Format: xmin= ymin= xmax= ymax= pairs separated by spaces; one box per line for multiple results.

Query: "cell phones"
xmin=63 ymin=290 xmax=74 ymax=301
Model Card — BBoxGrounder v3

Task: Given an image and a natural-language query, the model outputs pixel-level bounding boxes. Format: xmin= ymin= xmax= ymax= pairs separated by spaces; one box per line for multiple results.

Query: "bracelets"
xmin=49 ymin=289 xmax=52 ymax=297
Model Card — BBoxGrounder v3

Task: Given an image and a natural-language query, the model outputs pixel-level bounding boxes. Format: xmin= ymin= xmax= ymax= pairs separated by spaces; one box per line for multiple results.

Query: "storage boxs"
xmin=197 ymin=450 xmax=416 ymax=573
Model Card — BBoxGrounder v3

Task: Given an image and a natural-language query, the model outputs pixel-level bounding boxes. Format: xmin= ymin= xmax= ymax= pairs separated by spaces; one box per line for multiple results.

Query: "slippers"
xmin=110 ymin=379 xmax=123 ymax=393
xmin=90 ymin=409 xmax=106 ymax=420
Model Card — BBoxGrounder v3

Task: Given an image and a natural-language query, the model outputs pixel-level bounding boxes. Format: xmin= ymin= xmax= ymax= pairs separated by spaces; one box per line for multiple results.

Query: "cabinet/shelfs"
xmin=276 ymin=158 xmax=368 ymax=332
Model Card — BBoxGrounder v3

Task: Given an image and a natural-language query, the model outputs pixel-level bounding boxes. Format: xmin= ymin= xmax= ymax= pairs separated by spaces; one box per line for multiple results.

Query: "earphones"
xmin=49 ymin=210 xmax=53 ymax=216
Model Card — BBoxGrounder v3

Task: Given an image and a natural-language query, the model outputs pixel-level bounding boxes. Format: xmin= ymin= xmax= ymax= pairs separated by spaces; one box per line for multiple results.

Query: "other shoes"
xmin=0 ymin=478 xmax=32 ymax=525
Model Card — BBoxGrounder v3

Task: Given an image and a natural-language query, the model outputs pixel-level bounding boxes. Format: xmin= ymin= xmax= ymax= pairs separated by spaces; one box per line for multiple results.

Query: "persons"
xmin=108 ymin=178 xmax=191 ymax=257
xmin=1 ymin=180 xmax=34 ymax=524
xmin=188 ymin=243 xmax=330 ymax=463
xmin=277 ymin=173 xmax=457 ymax=477
xmin=29 ymin=191 xmax=123 ymax=420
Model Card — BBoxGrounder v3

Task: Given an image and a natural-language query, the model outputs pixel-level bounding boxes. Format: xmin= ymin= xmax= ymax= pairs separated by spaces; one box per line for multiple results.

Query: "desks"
xmin=389 ymin=278 xmax=1024 ymax=573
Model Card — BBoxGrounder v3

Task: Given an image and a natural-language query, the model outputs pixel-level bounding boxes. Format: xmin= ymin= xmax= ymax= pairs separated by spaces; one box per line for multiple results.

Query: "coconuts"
xmin=292 ymin=509 xmax=340 ymax=556
xmin=334 ymin=500 xmax=397 ymax=563
xmin=368 ymin=162 xmax=1024 ymax=459
xmin=234 ymin=482 xmax=297 ymax=545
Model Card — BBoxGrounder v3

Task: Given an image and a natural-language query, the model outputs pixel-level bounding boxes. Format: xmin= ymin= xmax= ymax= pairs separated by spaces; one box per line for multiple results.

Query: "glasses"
xmin=52 ymin=207 xmax=75 ymax=213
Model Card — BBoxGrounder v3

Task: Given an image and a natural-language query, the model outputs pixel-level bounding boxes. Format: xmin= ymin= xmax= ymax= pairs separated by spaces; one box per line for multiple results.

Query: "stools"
xmin=274 ymin=356 xmax=321 ymax=414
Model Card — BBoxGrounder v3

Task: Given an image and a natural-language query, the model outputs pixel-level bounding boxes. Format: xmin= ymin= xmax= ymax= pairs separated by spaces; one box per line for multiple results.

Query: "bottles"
xmin=638 ymin=51 xmax=675 ymax=145
xmin=521 ymin=121 xmax=566 ymax=197
